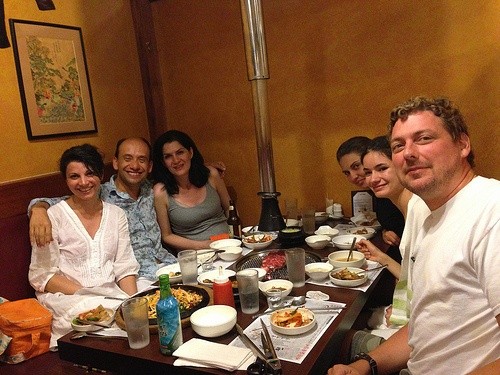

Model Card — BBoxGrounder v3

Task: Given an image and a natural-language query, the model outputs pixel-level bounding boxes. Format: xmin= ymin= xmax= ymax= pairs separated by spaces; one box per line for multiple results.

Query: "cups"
xmin=285 ymin=248 xmax=305 ymax=287
xmin=284 ymin=199 xmax=297 ymax=223
xmin=122 ymin=297 xmax=150 ymax=349
xmin=178 ymin=249 xmax=198 ymax=284
xmin=236 ymin=269 xmax=259 ymax=315
xmin=302 ymin=207 xmax=315 ymax=234
xmin=266 ymin=289 xmax=282 ymax=309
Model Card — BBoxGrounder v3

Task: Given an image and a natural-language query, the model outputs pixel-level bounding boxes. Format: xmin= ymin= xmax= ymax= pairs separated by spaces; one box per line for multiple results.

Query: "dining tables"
xmin=55 ymin=217 xmax=395 ymax=375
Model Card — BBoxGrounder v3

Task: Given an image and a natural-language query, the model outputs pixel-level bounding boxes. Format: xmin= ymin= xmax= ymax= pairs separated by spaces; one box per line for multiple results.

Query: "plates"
xmin=270 ymin=308 xmax=316 ymax=335
xmin=115 ymin=284 xmax=214 ymax=333
xmin=196 ymin=268 xmax=236 ymax=285
xmin=329 ymin=267 xmax=368 ymax=287
xmin=345 ymin=227 xmax=375 ymax=235
xmin=192 ymin=249 xmax=217 ymax=263
xmin=71 ymin=308 xmax=115 ymax=332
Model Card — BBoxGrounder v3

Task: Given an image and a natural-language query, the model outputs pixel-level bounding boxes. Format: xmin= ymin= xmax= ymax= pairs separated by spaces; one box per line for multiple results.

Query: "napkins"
xmin=171 ymin=336 xmax=257 ymax=373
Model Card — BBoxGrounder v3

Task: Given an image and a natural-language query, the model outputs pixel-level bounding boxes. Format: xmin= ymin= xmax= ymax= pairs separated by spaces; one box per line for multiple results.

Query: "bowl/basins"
xmin=242 ymin=225 xmax=272 ymax=249
xmin=238 ymin=268 xmax=267 ymax=281
xmin=315 ymin=212 xmax=329 ymax=222
xmin=279 ymin=226 xmax=304 ymax=242
xmin=190 ymin=304 xmax=237 ymax=337
xmin=332 ymin=235 xmax=367 ymax=249
xmin=305 ymin=262 xmax=334 ymax=281
xmin=328 ymin=250 xmax=366 ymax=268
xmin=155 ymin=262 xmax=202 ymax=282
xmin=259 ymin=279 xmax=294 ymax=297
xmin=305 ymin=225 xmax=339 ymax=249
xmin=210 ymin=238 xmax=243 ymax=262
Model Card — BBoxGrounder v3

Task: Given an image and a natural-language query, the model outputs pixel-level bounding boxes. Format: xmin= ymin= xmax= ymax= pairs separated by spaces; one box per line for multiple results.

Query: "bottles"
xmin=156 ymin=274 xmax=183 ymax=355
xmin=227 ymin=200 xmax=242 ymax=241
xmin=214 ymin=266 xmax=235 ymax=308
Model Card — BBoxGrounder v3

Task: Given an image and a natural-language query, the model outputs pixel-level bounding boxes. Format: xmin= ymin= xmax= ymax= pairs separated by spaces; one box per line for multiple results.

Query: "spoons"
xmin=198 ymin=250 xmax=225 ymax=268
xmin=69 ymin=332 xmax=128 ymax=339
xmin=251 ymin=295 xmax=305 ymax=318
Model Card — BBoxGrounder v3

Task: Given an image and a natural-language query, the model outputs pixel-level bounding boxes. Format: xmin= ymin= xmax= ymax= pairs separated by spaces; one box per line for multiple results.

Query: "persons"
xmin=151 ymin=129 xmax=240 ymax=257
xmin=355 ymin=136 xmax=431 ymax=340
xmin=336 ymin=137 xmax=405 ymax=311
xmin=28 ymin=144 xmax=140 ymax=336
xmin=28 ymin=136 xmax=227 ymax=293
xmin=328 ymin=94 xmax=500 ymax=375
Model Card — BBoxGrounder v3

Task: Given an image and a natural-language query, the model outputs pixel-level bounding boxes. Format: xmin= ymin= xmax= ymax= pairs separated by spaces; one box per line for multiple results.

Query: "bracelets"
xmin=351 ymin=351 xmax=378 ymax=375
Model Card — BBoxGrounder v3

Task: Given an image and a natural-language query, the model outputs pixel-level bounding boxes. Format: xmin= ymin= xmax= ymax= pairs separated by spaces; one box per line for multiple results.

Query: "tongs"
xmin=236 ymin=318 xmax=282 ymax=375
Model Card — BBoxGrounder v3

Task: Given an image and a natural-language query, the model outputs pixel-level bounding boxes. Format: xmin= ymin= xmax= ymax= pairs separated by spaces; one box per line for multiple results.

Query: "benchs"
xmin=0 ymin=161 xmax=117 ymax=375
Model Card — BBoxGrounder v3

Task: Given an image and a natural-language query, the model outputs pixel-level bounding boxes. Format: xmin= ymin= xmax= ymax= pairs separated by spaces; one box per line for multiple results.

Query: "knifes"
xmin=77 ymin=320 xmax=111 ymax=328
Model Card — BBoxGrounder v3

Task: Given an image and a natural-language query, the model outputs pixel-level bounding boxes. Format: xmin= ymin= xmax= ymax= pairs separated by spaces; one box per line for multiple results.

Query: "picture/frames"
xmin=9 ymin=18 xmax=100 ymax=142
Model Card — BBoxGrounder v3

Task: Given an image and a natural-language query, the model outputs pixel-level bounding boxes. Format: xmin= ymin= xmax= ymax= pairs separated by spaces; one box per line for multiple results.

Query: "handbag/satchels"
xmin=0 ymin=298 xmax=52 ymax=364
